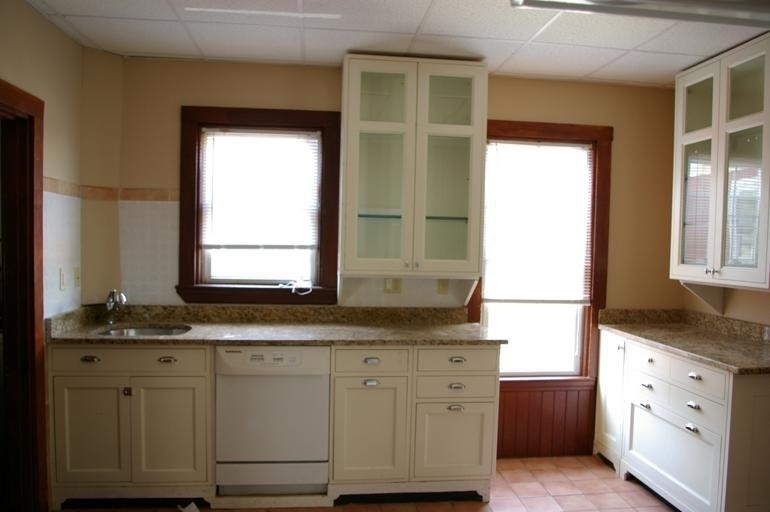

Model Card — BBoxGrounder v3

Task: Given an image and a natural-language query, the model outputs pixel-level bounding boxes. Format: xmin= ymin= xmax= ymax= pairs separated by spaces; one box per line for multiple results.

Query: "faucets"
xmin=105 ymin=288 xmax=127 ymax=322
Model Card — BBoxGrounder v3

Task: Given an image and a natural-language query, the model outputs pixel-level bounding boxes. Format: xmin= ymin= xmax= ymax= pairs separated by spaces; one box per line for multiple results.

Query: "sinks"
xmin=100 ymin=324 xmax=192 ymax=337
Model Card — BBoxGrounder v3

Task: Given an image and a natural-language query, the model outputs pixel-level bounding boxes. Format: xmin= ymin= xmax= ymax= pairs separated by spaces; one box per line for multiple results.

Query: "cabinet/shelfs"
xmin=336 ymin=54 xmax=489 ymax=309
xmin=669 ymin=30 xmax=770 ymax=293
xmin=592 ymin=331 xmax=770 ymax=512
